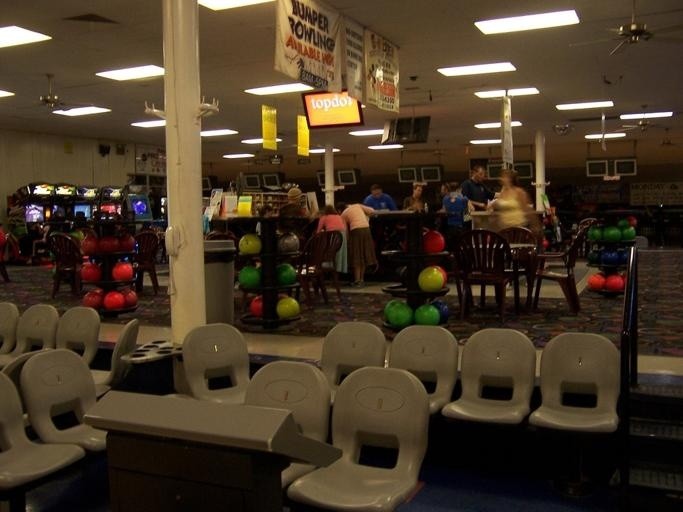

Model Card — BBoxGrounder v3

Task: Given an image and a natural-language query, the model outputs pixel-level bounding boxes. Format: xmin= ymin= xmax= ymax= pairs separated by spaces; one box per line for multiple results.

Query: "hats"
xmin=287 ymin=187 xmax=307 ymax=200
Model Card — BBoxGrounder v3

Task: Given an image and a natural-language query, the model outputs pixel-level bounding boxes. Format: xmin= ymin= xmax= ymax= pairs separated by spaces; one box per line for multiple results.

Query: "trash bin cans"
xmin=204 ymin=240 xmax=237 ymax=326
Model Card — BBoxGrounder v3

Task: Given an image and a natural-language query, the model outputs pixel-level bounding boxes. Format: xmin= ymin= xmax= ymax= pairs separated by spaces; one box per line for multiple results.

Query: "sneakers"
xmin=348 ymin=280 xmax=366 ymax=288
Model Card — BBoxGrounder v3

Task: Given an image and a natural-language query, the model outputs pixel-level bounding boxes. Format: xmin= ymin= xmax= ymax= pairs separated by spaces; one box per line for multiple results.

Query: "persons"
xmin=362 ymin=182 xmax=399 ymax=211
xmin=4 ymin=190 xmax=30 ymax=258
xmin=487 ymin=166 xmax=544 ymax=249
xmin=277 ymin=185 xmax=317 ymax=220
xmin=400 ymin=181 xmax=429 ymax=210
xmin=335 ymin=201 xmax=382 ymax=286
xmin=437 ymin=180 xmax=475 ymax=231
xmin=459 ymin=163 xmax=495 ymax=213
xmin=313 ymin=203 xmax=348 ymax=286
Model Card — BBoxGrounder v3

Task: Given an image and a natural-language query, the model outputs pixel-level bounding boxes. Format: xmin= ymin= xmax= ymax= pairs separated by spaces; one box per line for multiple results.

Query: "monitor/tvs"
xmin=301 ymin=88 xmax=365 ymax=130
xmin=74 ymin=203 xmax=91 ymax=218
xmin=101 ymin=202 xmax=122 ymax=218
xmin=201 ymin=177 xmax=214 ymax=190
xmin=315 ymin=169 xmax=359 ymax=186
xmin=243 ymin=172 xmax=282 ymax=188
xmin=585 ymin=158 xmax=638 ymax=177
xmin=486 ymin=161 xmax=533 ymax=181
xmin=25 ymin=203 xmax=45 ymax=222
xmin=130 ymin=197 xmax=149 ymax=215
xmin=397 ymin=165 xmax=443 ymax=183
xmin=51 ymin=204 xmax=66 ymax=217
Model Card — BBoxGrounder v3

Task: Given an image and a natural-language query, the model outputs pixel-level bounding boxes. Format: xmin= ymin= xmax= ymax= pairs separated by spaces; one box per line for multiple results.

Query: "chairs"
xmin=439 ymin=328 xmax=537 ymax=425
xmin=306 ymin=229 xmax=343 ymax=304
xmin=1 ymin=373 xmax=87 ymax=512
xmin=0 ymin=301 xmax=141 ymax=429
xmin=19 ymin=349 xmax=108 ymax=452
xmin=134 ymin=232 xmax=159 ymax=294
xmin=525 ymin=331 xmax=622 ymax=435
xmin=319 ymin=321 xmax=387 ymax=405
xmin=246 ymin=361 xmax=332 ymax=490
xmin=183 ymin=325 xmax=252 ymax=406
xmin=45 ymin=232 xmax=81 ymax=299
xmin=286 ymin=366 xmax=430 ymax=510
xmin=451 ymin=223 xmax=585 ymax=322
xmin=384 ymin=325 xmax=460 ymax=416
xmin=294 ymin=229 xmax=329 ymax=303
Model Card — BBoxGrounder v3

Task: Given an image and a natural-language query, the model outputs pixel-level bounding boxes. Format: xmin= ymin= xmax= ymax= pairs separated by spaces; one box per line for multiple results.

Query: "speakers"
xmin=381 ymin=115 xmax=431 ymax=145
xmin=98 ymin=144 xmax=111 ymax=154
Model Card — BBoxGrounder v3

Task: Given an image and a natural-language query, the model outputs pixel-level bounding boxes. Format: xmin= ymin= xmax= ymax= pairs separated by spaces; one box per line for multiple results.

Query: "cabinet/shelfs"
xmin=379 ymin=210 xmax=452 ymax=332
xmin=81 ymin=218 xmax=139 ymax=317
xmin=584 ymin=209 xmax=643 ymax=299
xmin=226 ymin=214 xmax=308 ymax=327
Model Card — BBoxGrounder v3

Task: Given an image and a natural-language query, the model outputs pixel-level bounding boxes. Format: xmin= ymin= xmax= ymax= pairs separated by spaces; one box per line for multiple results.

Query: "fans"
xmin=8 ymin=73 xmax=99 ymax=111
xmin=567 ymin=1 xmax=682 ymax=58
xmin=616 ymin=104 xmax=667 ymax=133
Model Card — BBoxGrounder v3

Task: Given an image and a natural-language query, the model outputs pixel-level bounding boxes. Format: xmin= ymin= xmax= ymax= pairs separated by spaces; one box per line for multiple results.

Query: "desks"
xmin=83 ymin=390 xmax=345 ymax=512
xmin=96 ymin=322 xmax=683 ymax=369
